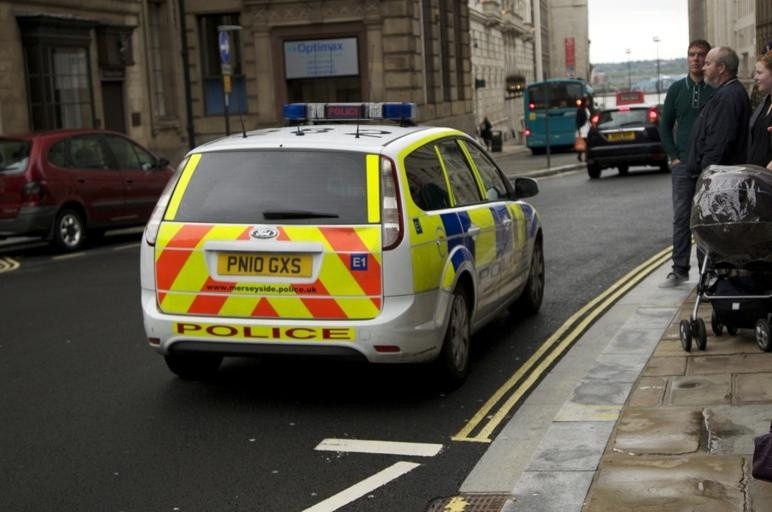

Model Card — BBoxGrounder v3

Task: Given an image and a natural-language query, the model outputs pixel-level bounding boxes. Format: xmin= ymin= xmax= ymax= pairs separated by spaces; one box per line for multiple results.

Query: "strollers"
xmin=678 ymin=163 xmax=772 ymax=354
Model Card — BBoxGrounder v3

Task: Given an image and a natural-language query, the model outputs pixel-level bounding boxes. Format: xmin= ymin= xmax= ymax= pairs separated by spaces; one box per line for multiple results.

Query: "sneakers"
xmin=658 ymin=270 xmax=689 ymax=288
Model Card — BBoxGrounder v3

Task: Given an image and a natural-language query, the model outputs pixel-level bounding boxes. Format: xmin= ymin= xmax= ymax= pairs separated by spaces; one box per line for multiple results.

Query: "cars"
xmin=0 ymin=128 xmax=177 ymax=254
xmin=139 ymin=99 xmax=547 ymax=399
xmin=585 ymin=104 xmax=670 ymax=179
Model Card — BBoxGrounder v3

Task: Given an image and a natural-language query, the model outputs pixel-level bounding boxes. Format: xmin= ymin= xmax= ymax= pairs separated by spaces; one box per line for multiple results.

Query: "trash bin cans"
xmin=491 ymin=130 xmax=502 ymax=151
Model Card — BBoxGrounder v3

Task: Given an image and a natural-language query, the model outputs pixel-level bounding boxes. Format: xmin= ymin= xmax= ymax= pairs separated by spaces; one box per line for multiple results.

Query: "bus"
xmin=523 ymin=78 xmax=594 ymax=155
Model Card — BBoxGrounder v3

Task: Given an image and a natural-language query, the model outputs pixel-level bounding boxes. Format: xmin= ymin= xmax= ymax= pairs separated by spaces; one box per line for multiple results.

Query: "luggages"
xmin=492 ymin=136 xmax=502 ymax=152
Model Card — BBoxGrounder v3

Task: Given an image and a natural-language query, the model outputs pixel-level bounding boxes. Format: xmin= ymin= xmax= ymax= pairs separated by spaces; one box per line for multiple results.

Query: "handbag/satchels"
xmin=480 ymin=122 xmax=486 ymax=130
xmin=574 ymin=136 xmax=588 ymax=151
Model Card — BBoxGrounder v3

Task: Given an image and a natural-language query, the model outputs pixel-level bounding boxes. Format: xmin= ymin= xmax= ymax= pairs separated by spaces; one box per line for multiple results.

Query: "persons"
xmin=575 ymin=96 xmax=591 ymax=162
xmin=660 ymin=41 xmax=772 ymax=288
xmin=479 ymin=117 xmax=492 ymax=151
